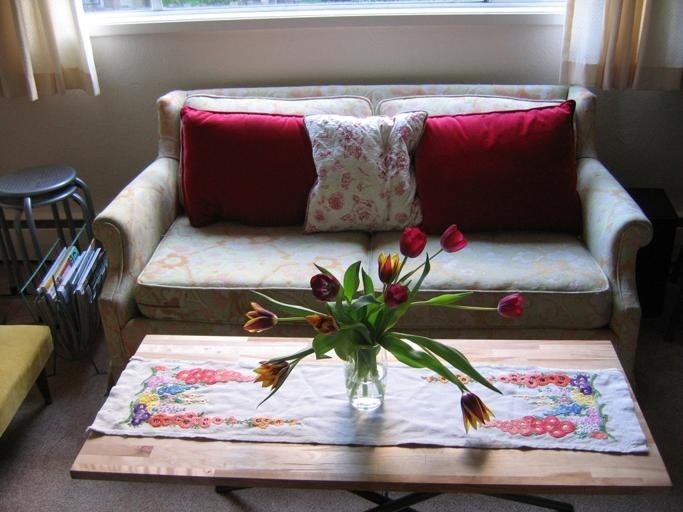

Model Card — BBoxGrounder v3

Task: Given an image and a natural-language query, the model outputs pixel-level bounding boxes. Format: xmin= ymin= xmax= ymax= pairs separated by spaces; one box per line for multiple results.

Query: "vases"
xmin=346 ymin=344 xmax=388 ymax=411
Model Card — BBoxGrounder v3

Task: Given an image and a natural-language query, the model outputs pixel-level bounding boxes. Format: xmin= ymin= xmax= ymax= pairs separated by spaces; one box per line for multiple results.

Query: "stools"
xmin=0 ymin=164 xmax=93 ymax=297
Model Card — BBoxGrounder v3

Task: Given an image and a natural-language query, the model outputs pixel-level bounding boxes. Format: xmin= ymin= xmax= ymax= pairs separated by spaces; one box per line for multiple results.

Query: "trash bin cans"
xmin=625 ymin=187 xmax=683 ymax=317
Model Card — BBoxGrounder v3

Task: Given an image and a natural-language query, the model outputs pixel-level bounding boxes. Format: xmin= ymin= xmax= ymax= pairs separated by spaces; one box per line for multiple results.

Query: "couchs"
xmin=0 ymin=323 xmax=55 ymax=439
xmin=90 ymin=85 xmax=654 ymax=391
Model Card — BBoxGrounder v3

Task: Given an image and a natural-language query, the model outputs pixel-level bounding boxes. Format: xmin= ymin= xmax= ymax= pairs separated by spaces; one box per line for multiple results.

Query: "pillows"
xmin=410 ymin=99 xmax=582 ymax=235
xmin=178 ymin=105 xmax=308 ymax=229
xmin=302 ymin=109 xmax=428 ymax=235
xmin=376 ymin=94 xmax=567 ymax=115
xmin=184 ymin=94 xmax=375 ymax=118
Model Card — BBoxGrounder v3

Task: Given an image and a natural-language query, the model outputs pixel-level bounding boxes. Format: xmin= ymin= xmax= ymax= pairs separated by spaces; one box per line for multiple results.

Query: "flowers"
xmin=240 ymin=225 xmax=525 ymax=434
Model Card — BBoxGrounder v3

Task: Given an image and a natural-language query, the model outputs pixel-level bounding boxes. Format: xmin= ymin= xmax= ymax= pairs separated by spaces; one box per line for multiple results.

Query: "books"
xmin=32 ymin=237 xmax=110 ymax=353
